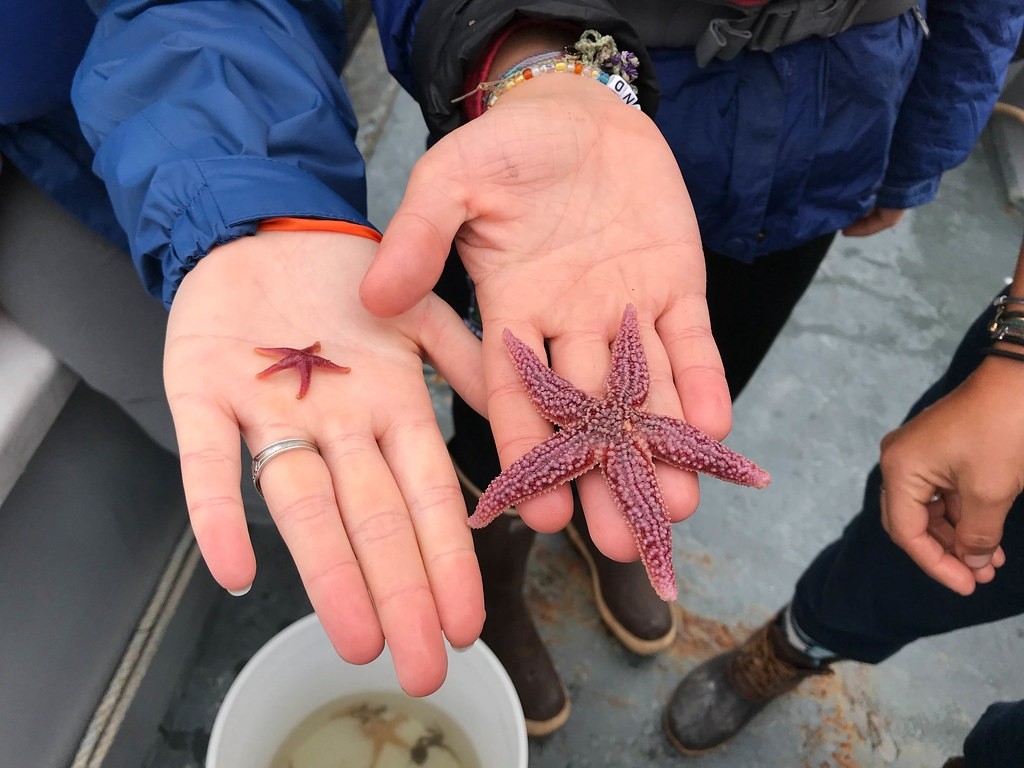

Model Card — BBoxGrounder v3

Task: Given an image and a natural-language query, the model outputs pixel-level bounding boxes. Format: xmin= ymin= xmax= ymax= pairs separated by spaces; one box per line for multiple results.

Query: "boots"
xmin=564 ymin=498 xmax=679 ymax=657
xmin=437 ymin=433 xmax=572 ymax=737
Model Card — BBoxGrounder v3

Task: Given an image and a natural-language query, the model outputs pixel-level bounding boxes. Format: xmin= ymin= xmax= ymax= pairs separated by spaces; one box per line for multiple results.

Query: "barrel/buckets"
xmin=206 ymin=610 xmax=529 ymax=768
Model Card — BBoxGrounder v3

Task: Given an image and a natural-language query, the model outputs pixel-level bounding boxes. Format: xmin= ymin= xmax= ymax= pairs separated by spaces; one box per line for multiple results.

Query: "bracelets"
xmin=450 ymin=29 xmax=647 ymax=111
xmin=985 ymin=294 xmax=1024 ymax=362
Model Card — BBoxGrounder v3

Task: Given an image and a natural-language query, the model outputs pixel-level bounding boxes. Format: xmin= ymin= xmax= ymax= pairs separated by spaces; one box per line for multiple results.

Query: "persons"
xmin=361 ymin=0 xmax=1020 ymax=733
xmin=658 ymin=242 xmax=1024 ymax=768
xmin=0 ymin=1 xmax=488 ymax=699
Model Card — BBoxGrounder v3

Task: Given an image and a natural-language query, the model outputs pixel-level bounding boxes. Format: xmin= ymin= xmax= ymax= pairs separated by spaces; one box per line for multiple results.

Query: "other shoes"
xmin=662 ymin=607 xmax=835 ymax=758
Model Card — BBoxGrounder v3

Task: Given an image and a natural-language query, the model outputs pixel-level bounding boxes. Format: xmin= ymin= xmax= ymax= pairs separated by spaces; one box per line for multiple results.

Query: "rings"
xmin=245 ymin=438 xmax=329 ymax=500
